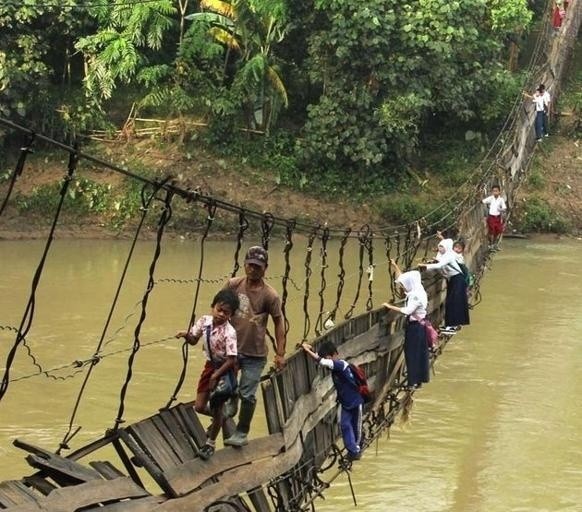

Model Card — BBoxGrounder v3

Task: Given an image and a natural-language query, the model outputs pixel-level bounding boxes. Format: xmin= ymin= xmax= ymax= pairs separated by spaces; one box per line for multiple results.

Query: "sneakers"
xmin=341 ymin=450 xmax=364 ymax=462
xmin=535 ymin=133 xmax=553 ymax=143
xmin=197 ymin=402 xmax=257 ymax=460
xmin=439 ymin=324 xmax=459 ymax=335
xmin=488 ymin=244 xmax=503 ymax=252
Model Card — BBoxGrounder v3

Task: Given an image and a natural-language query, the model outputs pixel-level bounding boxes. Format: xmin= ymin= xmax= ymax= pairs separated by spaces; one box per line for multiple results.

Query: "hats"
xmin=244 ymin=245 xmax=269 ymax=268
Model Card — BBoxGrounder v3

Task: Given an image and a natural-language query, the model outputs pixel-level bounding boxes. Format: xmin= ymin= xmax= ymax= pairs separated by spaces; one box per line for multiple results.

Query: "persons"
xmin=548 ymin=0 xmax=571 ymax=37
xmin=530 ymin=90 xmax=544 ymax=143
xmin=520 ymin=84 xmax=552 ymax=138
xmin=451 ymin=240 xmax=469 ymax=286
xmin=174 ymin=289 xmax=240 ymax=462
xmin=381 ymin=258 xmax=429 ymax=389
xmin=211 ymin=246 xmax=287 ymax=447
xmin=479 ymin=184 xmax=507 ymax=252
xmin=297 ymin=337 xmax=369 ymax=461
xmin=417 ymin=231 xmax=470 ymax=335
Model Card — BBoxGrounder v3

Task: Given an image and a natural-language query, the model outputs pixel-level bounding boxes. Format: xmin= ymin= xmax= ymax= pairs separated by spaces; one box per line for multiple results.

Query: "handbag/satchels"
xmin=422 ymin=318 xmax=438 ymax=346
xmin=207 ymin=369 xmax=234 ymax=402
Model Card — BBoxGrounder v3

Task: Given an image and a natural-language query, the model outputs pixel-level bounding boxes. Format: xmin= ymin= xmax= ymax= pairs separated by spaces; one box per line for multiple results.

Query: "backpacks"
xmin=334 ymin=362 xmax=372 ymax=403
xmin=447 ymin=260 xmax=470 ymax=287
